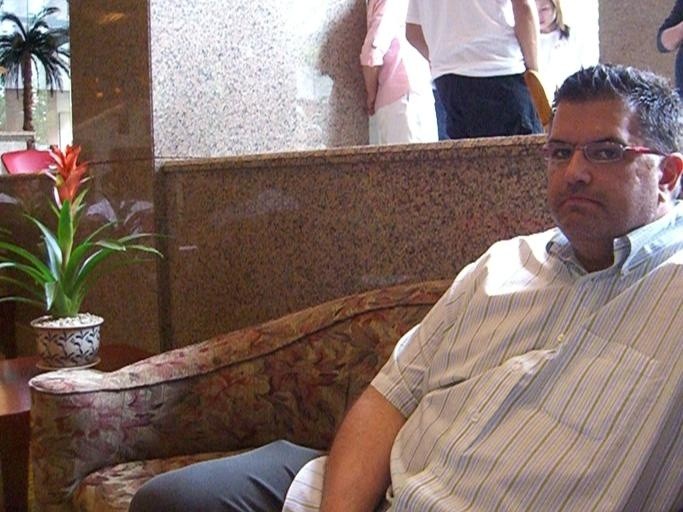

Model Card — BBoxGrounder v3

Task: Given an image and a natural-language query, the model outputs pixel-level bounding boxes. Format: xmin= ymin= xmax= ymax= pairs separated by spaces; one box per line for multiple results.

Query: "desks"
xmin=0 ymin=339 xmax=154 ymax=510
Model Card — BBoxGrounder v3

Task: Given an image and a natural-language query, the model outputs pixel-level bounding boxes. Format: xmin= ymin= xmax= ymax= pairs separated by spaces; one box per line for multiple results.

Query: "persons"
xmin=404 ymin=0 xmax=545 ymax=139
xmin=128 ymin=67 xmax=682 ymax=510
xmin=527 ymin=0 xmax=579 ymax=99
xmin=357 ymin=2 xmax=439 ymax=146
xmin=656 ymin=0 xmax=683 ymax=101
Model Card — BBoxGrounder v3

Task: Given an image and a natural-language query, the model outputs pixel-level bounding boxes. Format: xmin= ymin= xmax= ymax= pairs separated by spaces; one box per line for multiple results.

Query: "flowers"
xmin=0 ymin=140 xmax=178 ymax=319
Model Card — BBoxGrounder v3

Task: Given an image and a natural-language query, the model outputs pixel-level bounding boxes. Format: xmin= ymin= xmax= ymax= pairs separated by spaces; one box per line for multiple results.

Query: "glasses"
xmin=542 ymin=139 xmax=672 ymax=164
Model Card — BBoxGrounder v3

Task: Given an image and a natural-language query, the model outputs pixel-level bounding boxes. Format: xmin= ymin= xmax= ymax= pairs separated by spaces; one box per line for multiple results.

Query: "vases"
xmin=27 ymin=311 xmax=105 ymax=366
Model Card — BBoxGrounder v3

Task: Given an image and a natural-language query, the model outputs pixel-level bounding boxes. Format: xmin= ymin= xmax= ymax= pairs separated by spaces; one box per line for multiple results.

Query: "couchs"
xmin=23 ymin=277 xmax=454 ymax=511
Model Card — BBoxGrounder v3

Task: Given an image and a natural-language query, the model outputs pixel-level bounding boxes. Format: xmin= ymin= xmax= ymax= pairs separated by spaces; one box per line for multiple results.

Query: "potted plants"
xmin=0 ymin=1 xmax=71 ymax=174
